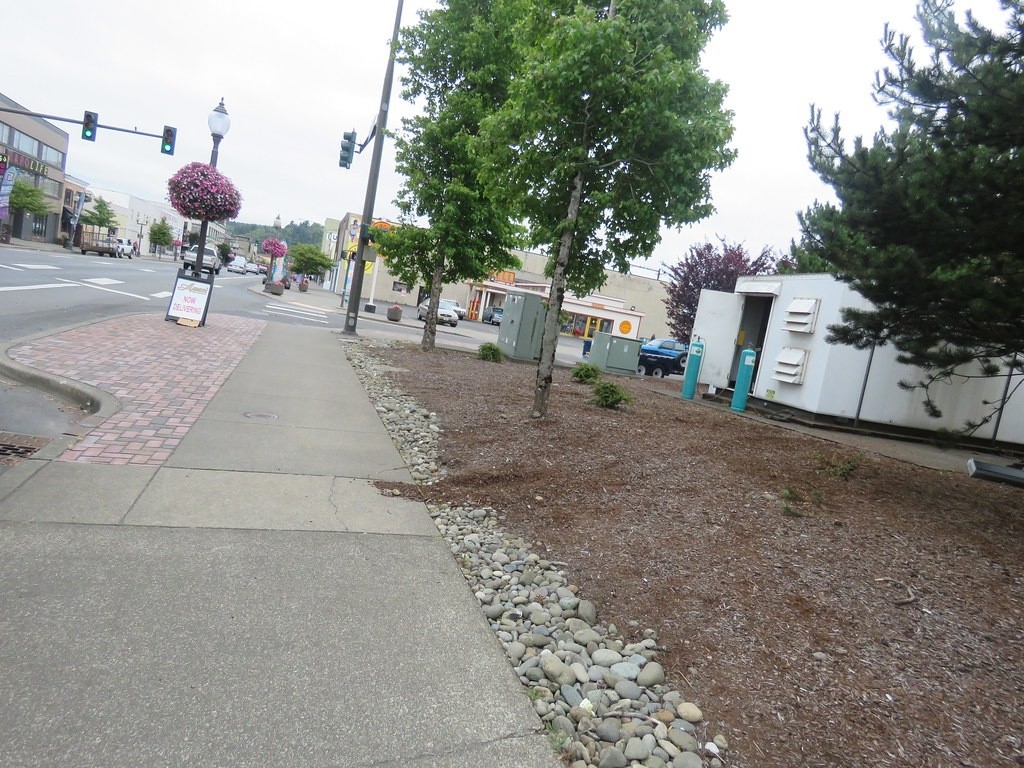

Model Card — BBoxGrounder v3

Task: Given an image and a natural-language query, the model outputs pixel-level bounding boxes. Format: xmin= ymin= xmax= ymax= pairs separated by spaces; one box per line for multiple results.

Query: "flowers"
xmin=270 ymin=280 xmax=285 ymax=288
xmin=392 ymin=305 xmax=401 ymax=311
xmin=299 ymin=280 xmax=309 ymax=286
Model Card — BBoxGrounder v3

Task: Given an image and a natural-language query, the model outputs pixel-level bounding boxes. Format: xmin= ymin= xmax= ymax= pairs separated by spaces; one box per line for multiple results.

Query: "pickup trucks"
xmin=443 ymin=299 xmax=466 ymax=320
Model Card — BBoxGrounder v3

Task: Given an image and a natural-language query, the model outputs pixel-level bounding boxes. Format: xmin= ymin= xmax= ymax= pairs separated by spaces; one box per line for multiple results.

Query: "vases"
xmin=298 ymin=285 xmax=308 ymax=293
xmin=271 ymin=285 xmax=284 ymax=295
xmin=387 ymin=308 xmax=403 ymax=322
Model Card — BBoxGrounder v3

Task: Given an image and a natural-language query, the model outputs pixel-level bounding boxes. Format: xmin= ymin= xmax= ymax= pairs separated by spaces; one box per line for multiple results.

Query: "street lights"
xmin=264 ymin=214 xmax=282 ymax=292
xmin=192 ymin=96 xmax=230 ymax=277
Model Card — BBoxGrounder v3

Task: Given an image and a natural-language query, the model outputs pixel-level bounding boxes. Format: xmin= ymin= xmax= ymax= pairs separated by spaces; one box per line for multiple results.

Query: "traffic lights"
xmin=338 ymin=131 xmax=357 ymax=169
xmin=160 ymin=125 xmax=177 ymax=156
xmin=81 ymin=110 xmax=98 ymax=142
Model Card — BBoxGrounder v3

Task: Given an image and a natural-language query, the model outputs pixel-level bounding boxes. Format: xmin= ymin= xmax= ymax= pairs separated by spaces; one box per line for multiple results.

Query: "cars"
xmin=641 ymin=338 xmax=688 ymax=357
xmin=417 ymin=298 xmax=458 ymax=327
xmin=184 ymin=245 xmax=222 ymax=274
xmin=246 ymin=263 xmax=260 ymax=275
xmin=99 ymin=237 xmax=135 ymax=260
xmin=227 ymin=261 xmax=246 ymax=275
xmin=482 ymin=307 xmax=503 ymax=325
